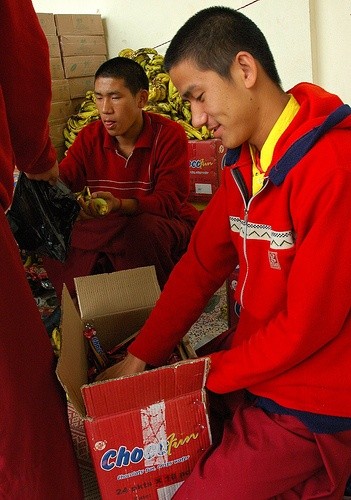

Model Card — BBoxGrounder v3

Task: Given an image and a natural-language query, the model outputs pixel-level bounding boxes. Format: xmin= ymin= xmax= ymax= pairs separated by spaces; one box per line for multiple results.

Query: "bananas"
xmin=82 ymin=198 xmax=108 ymax=216
xmin=63 ymin=46 xmax=214 ymax=155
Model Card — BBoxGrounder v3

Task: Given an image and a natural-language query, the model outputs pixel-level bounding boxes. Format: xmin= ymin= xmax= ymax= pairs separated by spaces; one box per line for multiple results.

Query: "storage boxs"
xmin=188 ymin=138 xmax=227 ymax=204
xmin=35 ymin=13 xmax=107 ymax=164
xmin=55 ymin=265 xmax=212 ymax=500
xmin=226 ymin=267 xmax=240 ymax=328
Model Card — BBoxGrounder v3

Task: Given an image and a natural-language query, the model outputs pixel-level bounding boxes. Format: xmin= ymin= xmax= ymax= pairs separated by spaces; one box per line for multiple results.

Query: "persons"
xmin=0 ymin=0 xmax=83 ymax=499
xmin=93 ymin=4 xmax=351 ymax=499
xmin=58 ymin=57 xmax=199 ymax=290
